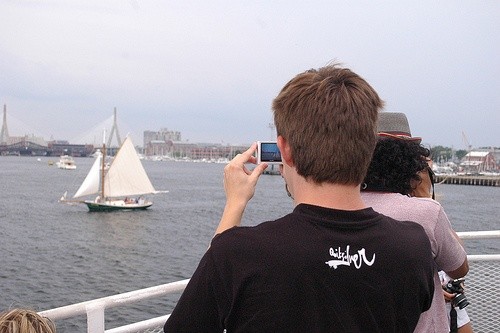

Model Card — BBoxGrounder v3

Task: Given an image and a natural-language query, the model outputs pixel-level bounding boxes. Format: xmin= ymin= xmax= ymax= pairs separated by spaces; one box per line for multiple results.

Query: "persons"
xmin=361 ymin=112 xmax=469 ymax=333
xmin=162 ymin=60 xmax=435 ymax=333
xmin=437 ymin=270 xmax=475 ymax=333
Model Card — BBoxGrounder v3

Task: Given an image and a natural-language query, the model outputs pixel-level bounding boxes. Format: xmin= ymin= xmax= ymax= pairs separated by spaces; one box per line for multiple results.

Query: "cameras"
xmin=255 ymin=141 xmax=284 ymax=165
xmin=442 ymin=276 xmax=469 ymax=310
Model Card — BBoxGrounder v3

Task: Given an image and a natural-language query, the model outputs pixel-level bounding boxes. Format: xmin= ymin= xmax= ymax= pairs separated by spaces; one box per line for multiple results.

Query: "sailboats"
xmin=56 ymin=128 xmax=170 ymax=212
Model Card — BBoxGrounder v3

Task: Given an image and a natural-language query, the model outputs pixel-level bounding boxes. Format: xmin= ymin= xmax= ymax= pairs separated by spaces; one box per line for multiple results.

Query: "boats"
xmin=56 ymin=154 xmax=77 ymax=170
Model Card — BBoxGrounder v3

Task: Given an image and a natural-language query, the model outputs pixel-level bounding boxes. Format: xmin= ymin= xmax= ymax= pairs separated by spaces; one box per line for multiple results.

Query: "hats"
xmin=376 ymin=112 xmax=422 ymax=145
